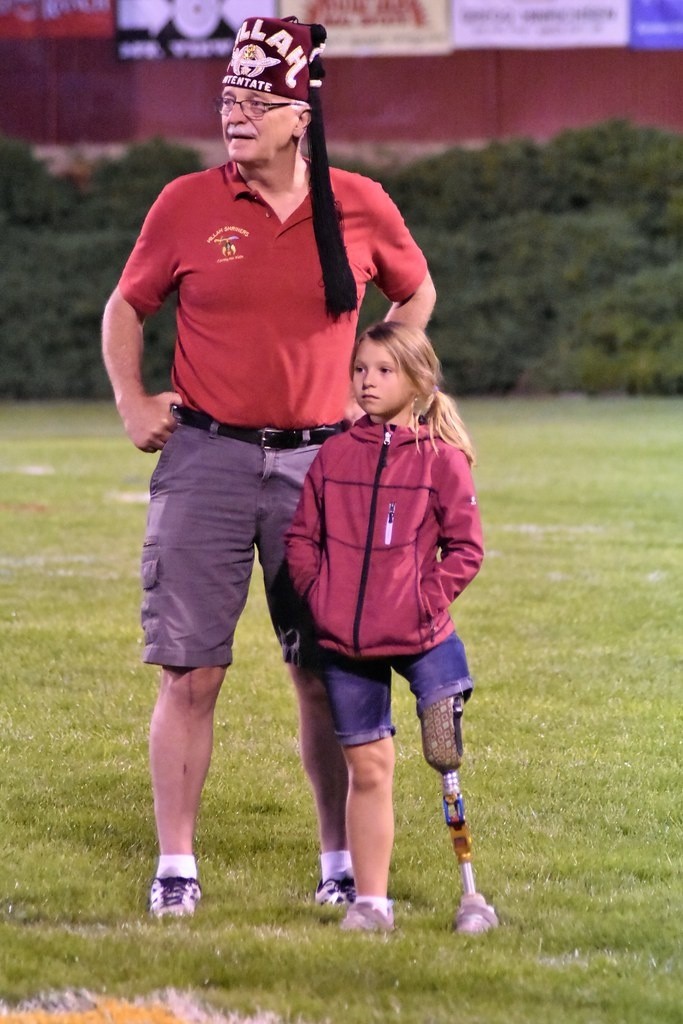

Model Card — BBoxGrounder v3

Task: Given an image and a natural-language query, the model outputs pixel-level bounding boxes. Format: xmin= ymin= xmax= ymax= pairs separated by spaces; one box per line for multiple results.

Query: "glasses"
xmin=216 ymin=95 xmax=306 ymax=120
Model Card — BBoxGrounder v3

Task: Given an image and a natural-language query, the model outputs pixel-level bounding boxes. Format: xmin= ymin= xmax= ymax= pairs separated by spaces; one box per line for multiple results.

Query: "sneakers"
xmin=315 ymin=876 xmax=356 ymax=908
xmin=454 ymin=892 xmax=499 ymax=934
xmin=149 ymin=874 xmax=202 ymax=921
xmin=339 ymin=900 xmax=394 ymax=932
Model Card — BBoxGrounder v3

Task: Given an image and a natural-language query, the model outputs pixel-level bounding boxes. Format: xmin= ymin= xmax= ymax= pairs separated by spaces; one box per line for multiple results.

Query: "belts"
xmin=170 ymin=402 xmax=345 ymax=451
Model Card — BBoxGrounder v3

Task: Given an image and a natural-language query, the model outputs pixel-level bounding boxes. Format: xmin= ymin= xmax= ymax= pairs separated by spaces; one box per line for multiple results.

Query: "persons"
xmin=102 ymin=18 xmax=437 ymax=921
xmin=285 ymin=320 xmax=499 ymax=939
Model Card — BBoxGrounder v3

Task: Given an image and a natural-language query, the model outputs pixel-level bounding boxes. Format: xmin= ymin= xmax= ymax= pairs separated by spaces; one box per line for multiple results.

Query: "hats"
xmin=221 ymin=16 xmax=326 ymax=102
